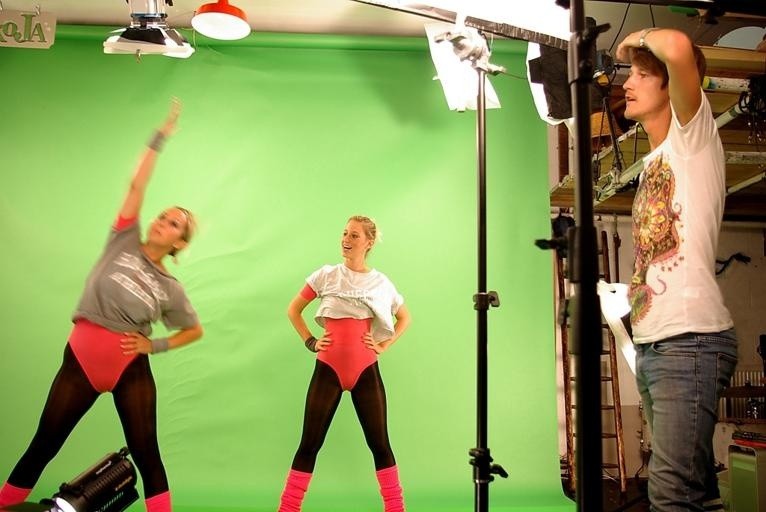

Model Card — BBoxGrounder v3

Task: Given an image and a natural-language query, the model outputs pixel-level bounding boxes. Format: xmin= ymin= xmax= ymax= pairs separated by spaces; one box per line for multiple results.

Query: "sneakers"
xmin=699 ymin=497 xmax=726 ymax=511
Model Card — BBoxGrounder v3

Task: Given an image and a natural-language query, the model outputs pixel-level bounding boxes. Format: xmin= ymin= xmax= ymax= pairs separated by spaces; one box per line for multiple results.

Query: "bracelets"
xmin=640 ymin=27 xmax=664 ymax=47
xmin=304 ymin=335 xmax=317 ymax=353
xmin=147 ymin=128 xmax=167 ymax=153
xmin=151 ymin=338 xmax=168 ymax=355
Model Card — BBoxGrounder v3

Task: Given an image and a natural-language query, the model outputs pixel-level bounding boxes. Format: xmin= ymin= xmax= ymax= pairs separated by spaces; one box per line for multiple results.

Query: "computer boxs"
xmin=728 ymin=444 xmax=766 ymax=512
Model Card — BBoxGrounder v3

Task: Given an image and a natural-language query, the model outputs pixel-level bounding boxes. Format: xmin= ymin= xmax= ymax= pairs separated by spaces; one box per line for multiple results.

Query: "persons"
xmin=276 ymin=214 xmax=410 ymax=512
xmin=0 ymin=101 xmax=204 ymax=512
xmin=612 ymin=24 xmax=738 ymax=512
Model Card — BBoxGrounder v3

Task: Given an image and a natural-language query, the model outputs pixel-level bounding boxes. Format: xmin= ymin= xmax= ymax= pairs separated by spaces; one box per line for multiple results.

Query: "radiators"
xmin=716 ymin=366 xmax=764 ymax=423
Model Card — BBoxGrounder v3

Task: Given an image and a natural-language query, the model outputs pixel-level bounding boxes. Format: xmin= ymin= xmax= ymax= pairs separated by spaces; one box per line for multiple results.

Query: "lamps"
xmin=44 ymin=446 xmax=144 ymax=510
xmin=103 ymin=0 xmax=196 ymax=58
xmin=191 ymin=0 xmax=252 ymax=43
